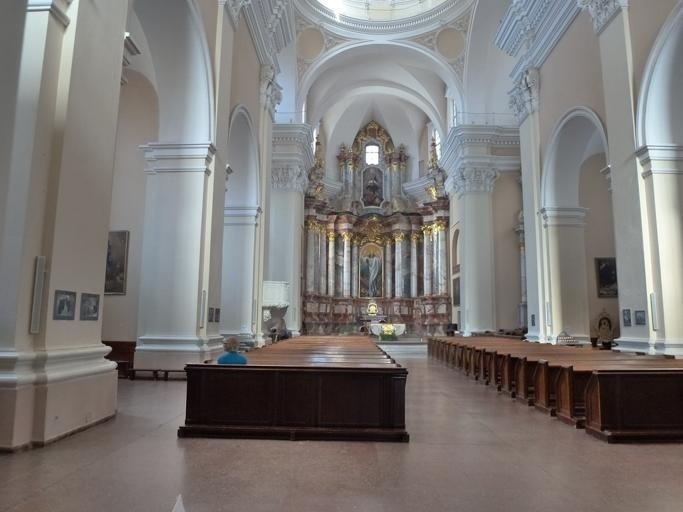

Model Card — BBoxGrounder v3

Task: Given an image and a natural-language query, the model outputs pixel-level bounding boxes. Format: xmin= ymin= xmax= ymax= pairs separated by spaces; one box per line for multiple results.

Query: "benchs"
xmin=177 ymin=336 xmax=412 ymax=443
xmin=425 ymin=334 xmax=682 ymax=442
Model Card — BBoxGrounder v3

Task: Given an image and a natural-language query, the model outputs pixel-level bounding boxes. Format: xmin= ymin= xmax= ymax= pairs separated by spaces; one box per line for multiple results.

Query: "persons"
xmin=216 ymin=336 xmax=248 ymax=364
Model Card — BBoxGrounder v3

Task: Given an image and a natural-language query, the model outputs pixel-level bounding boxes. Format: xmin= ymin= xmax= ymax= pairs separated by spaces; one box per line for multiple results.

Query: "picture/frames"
xmin=52 ymin=289 xmax=100 ymax=321
xmin=103 ymin=229 xmax=129 ymax=295
